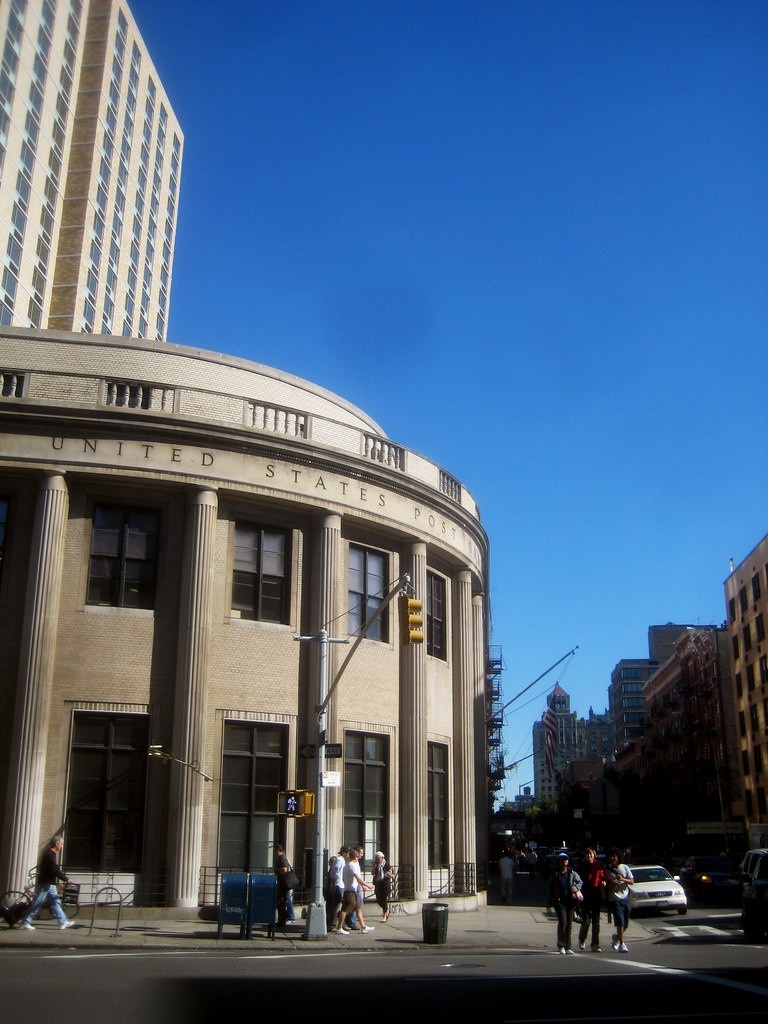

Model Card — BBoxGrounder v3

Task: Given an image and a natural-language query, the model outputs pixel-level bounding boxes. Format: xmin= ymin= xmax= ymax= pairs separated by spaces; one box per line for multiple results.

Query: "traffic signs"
xmin=298 ymin=743 xmax=342 ymax=759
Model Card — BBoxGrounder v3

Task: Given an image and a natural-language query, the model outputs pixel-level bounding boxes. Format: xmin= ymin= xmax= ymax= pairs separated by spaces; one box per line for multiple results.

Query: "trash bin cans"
xmin=420 ymin=901 xmax=449 ymax=944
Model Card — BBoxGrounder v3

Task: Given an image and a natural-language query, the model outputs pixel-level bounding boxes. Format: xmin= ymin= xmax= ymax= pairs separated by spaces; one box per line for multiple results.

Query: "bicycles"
xmin=25 ymin=875 xmax=80 ymax=920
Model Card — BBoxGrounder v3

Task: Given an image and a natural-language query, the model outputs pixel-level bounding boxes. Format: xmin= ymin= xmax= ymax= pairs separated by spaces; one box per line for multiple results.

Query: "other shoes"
xmin=20 ymin=923 xmax=35 ymax=930
xmin=557 ymin=947 xmax=566 ymax=955
xmin=579 ymin=937 xmax=585 ymax=949
xmin=507 ymin=900 xmax=512 ymax=904
xmin=591 ymin=944 xmax=602 ymax=952
xmin=289 ymin=920 xmax=296 ymax=924
xmin=60 ymin=920 xmax=75 ymax=930
xmin=619 ymin=943 xmax=629 ymax=952
xmin=502 ymin=895 xmax=506 ymax=903
xmin=613 ymin=934 xmax=620 ymax=951
xmin=327 ymin=910 xmax=390 ymax=935
xmin=566 ymin=949 xmax=574 ymax=955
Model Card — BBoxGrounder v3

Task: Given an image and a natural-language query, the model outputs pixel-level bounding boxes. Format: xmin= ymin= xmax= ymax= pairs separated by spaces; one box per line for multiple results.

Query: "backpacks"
xmin=322 ymin=873 xmax=331 ymax=901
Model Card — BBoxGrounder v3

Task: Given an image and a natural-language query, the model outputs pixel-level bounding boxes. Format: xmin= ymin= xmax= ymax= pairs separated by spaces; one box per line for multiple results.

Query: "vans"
xmin=742 ymin=849 xmax=768 ymax=943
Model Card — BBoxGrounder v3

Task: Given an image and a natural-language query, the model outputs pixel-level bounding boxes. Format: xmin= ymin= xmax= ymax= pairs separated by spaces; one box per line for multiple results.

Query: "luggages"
xmin=3 ymin=898 xmax=36 ymax=929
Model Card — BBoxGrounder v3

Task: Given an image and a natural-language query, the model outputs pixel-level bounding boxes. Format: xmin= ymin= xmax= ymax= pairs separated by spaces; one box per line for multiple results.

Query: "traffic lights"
xmin=398 ymin=596 xmax=423 ymax=645
xmin=277 ymin=790 xmax=315 ymax=817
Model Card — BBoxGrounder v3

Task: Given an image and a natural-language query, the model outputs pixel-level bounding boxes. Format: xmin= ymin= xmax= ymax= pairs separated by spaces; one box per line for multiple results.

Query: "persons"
xmin=329 ymin=846 xmax=375 ymax=935
xmin=498 ymin=852 xmax=521 ymax=904
xmin=371 ymin=851 xmax=392 ymax=922
xmin=274 ymin=843 xmax=296 ymax=925
xmin=546 ymin=853 xmax=584 ymax=954
xmin=525 ymin=848 xmax=537 ymax=880
xmin=577 ymin=848 xmax=606 ymax=952
xmin=605 ymin=846 xmax=634 ymax=953
xmin=17 ymin=836 xmax=75 ymax=931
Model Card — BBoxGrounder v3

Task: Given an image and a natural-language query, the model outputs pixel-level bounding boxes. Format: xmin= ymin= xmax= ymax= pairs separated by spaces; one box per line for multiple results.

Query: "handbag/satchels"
xmin=570 ymin=870 xmax=584 ymax=904
xmin=281 ymin=870 xmax=299 ymax=889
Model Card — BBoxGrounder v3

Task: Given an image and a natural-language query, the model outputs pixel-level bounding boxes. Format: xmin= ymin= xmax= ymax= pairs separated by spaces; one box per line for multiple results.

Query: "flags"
xmin=542 ymin=687 xmax=558 ymax=778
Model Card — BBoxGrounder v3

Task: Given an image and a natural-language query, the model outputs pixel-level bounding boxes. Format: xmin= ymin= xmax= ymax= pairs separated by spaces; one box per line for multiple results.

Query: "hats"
xmin=557 ymin=852 xmax=570 ymax=860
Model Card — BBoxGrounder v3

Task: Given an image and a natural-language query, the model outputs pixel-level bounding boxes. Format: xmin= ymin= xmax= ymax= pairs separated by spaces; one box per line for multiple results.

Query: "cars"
xmin=680 ymin=856 xmax=743 ymax=900
xmin=533 ymin=847 xmax=606 ymax=877
xmin=627 ymin=864 xmax=687 ymax=917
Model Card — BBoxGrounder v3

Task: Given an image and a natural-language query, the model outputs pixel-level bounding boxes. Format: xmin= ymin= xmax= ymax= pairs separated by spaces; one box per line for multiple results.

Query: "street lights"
xmin=693 ymin=730 xmax=729 ymax=853
